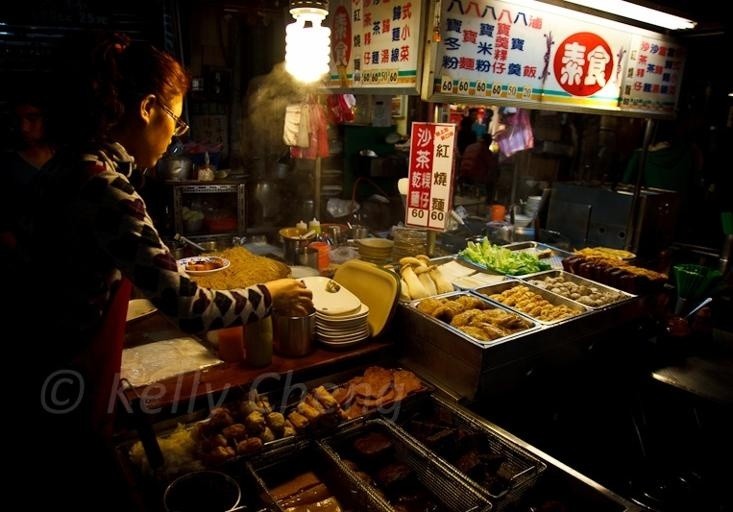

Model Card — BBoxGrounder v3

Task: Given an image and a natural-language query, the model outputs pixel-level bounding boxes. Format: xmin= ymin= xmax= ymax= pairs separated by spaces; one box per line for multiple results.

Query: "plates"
xmin=504 ymin=195 xmax=542 ymax=226
xmin=174 ymin=254 xmax=230 ymax=276
xmin=295 ymin=259 xmax=400 ymax=351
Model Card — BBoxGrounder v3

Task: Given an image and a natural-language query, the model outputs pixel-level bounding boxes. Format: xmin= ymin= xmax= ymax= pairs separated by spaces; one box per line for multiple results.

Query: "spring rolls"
xmin=289 ymin=385 xmax=336 ymax=427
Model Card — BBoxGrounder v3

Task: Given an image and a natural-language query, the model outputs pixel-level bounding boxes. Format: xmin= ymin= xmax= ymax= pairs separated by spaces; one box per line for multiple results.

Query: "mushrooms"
xmin=381 ymin=253 xmax=454 ymax=302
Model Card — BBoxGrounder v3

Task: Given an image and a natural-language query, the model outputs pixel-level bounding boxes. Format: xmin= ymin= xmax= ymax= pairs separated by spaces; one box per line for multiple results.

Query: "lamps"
xmin=283 ymin=0 xmax=331 ymax=87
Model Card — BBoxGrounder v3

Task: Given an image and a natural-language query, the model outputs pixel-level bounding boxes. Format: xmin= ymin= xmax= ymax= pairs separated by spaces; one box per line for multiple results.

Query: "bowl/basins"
xmin=357 ymin=237 xmax=393 ymax=264
xmin=278 ymin=226 xmax=315 ymax=255
xmin=320 ymin=223 xmax=346 ymax=233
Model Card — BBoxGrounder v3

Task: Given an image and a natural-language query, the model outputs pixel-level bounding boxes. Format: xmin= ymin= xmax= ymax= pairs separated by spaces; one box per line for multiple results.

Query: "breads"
xmin=420 ymin=246 xmax=670 ymax=344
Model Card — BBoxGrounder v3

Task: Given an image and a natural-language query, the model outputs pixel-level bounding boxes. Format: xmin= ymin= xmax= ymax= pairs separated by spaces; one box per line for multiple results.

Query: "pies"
xmin=331 ymin=364 xmax=425 ymax=418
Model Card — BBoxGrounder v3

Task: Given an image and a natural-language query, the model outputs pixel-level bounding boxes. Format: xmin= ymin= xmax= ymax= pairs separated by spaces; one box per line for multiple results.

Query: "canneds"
xmin=308 ymin=241 xmax=330 ymax=272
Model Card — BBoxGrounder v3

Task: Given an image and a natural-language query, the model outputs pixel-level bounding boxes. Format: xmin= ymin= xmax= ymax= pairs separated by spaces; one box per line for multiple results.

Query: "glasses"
xmin=155 ymin=100 xmax=190 ymax=137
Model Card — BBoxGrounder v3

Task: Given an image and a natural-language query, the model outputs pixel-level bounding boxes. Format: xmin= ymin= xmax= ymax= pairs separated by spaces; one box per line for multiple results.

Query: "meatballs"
xmin=191 ymin=399 xmax=297 ymax=458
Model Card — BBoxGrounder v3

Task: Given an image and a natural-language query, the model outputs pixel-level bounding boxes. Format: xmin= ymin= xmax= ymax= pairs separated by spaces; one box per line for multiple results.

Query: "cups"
xmin=279 ymin=309 xmax=313 ymax=357
xmin=490 ymin=204 xmax=507 ymax=222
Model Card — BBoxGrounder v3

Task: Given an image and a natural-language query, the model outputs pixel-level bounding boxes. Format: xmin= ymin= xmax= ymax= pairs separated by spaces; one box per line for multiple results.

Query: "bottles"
xmin=296 ymin=216 xmax=320 ymax=238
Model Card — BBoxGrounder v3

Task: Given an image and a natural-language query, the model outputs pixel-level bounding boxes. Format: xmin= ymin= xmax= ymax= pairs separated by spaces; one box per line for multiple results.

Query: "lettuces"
xmin=458 ymin=237 xmax=553 ymax=277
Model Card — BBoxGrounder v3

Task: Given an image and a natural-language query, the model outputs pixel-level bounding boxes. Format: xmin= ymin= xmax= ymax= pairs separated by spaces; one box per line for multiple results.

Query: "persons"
xmin=0 ymin=32 xmax=313 ymax=511
xmin=1 ymin=51 xmax=75 ymax=249
xmin=461 ymin=133 xmax=500 ymax=199
xmin=458 ymin=107 xmax=479 ymax=151
